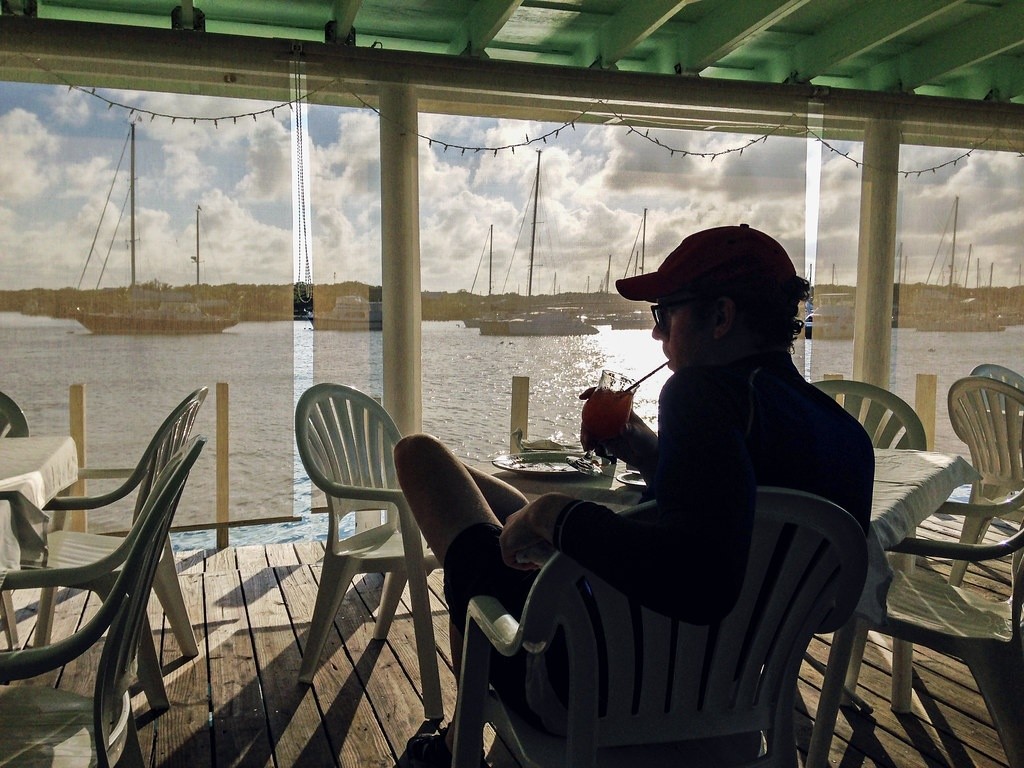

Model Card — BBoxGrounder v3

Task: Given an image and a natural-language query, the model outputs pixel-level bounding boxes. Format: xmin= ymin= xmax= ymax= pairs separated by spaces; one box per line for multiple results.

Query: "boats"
xmin=803 ymin=293 xmax=855 ymax=340
xmin=308 ymin=296 xmax=382 ymax=331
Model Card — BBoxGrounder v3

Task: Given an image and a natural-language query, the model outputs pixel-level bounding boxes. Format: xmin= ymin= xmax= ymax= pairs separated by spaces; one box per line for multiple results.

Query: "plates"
xmin=493 ymin=450 xmax=611 ymax=476
xmin=616 ymin=473 xmax=647 ymax=489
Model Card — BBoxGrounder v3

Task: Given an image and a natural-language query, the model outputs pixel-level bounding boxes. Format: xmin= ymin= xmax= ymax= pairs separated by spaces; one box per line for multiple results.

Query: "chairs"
xmin=0 ymin=435 xmax=211 ymax=768
xmin=296 ymin=383 xmax=468 ymax=724
xmin=946 ymin=377 xmax=1024 ymax=586
xmin=965 ymin=364 xmax=1024 ymax=493
xmin=810 ymin=380 xmax=928 ymax=712
xmin=447 ymin=489 xmax=868 ymax=768
xmin=837 ymin=487 xmax=1024 ymax=715
xmin=0 ymin=386 xmax=209 ymax=708
xmin=0 ymin=391 xmax=30 ymax=438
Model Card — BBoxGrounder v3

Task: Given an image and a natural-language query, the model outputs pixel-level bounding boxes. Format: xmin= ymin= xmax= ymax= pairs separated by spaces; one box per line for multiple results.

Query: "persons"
xmin=392 ymin=222 xmax=876 ymax=768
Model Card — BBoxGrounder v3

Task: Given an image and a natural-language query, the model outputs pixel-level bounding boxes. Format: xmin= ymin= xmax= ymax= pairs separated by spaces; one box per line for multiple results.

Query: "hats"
xmin=615 ymin=224 xmax=798 ymax=302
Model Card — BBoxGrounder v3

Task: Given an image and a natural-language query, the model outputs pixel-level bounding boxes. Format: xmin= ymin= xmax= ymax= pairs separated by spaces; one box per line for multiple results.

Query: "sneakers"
xmin=407 ymin=723 xmax=485 ymax=768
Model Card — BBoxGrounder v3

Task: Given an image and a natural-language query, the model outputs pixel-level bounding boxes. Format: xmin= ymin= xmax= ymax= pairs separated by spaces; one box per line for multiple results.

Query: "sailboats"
xmin=890 ymin=193 xmax=1024 ymax=332
xmin=463 ymin=149 xmax=657 ymax=336
xmin=73 ymin=120 xmax=238 ymax=333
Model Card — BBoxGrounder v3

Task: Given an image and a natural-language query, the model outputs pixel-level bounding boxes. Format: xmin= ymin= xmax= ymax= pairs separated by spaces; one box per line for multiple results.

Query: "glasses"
xmin=651 ymin=294 xmax=723 ymax=331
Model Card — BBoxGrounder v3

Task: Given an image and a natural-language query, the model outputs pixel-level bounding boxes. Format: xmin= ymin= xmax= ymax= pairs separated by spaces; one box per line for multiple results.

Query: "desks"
xmin=981 ymin=410 xmax=1024 ymax=555
xmin=488 ymin=447 xmax=983 ymax=768
xmin=0 ymin=436 xmax=78 ymax=650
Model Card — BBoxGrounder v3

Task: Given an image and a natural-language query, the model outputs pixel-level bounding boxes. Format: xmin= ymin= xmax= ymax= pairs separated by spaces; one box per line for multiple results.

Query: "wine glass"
xmin=565 ymin=370 xmax=639 ymax=475
